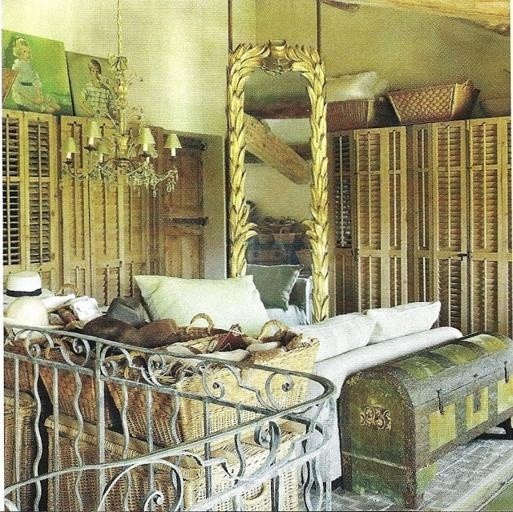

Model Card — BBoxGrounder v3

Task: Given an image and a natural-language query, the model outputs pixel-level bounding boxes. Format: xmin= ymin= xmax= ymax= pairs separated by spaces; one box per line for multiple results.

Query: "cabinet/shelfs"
xmin=326 ymin=116 xmax=511 ymax=339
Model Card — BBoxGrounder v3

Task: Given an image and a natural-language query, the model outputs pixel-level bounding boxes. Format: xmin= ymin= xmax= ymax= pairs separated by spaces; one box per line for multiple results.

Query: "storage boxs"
xmin=338 ymin=327 xmax=513 ymax=512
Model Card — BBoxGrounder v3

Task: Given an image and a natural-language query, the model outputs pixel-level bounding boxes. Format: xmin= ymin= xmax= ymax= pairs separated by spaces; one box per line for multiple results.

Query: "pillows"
xmin=367 ymin=300 xmax=443 ymax=344
xmin=241 ymin=264 xmax=306 ymax=312
xmin=285 ymin=310 xmax=374 ymax=363
xmin=133 ymin=273 xmax=272 ymax=341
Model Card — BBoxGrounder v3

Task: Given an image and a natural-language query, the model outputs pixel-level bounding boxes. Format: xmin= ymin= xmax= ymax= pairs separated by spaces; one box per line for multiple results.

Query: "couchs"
xmin=298 ymin=329 xmax=464 ymax=490
xmin=264 ymin=276 xmax=314 ymax=326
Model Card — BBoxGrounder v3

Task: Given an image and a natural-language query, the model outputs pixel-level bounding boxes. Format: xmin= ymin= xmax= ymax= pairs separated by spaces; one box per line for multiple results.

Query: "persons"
xmin=75 ymin=58 xmax=116 ymax=117
xmin=9 ymin=36 xmax=62 ymax=114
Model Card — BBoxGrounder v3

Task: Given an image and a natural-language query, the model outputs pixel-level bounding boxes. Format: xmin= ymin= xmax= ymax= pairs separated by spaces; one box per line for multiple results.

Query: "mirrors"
xmin=227 ymin=40 xmax=329 ymax=327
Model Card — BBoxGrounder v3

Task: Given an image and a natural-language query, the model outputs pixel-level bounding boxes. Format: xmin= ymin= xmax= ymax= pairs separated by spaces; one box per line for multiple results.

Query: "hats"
xmin=3 ymin=270 xmax=75 ymax=314
xmin=4 ymin=296 xmax=65 ymax=329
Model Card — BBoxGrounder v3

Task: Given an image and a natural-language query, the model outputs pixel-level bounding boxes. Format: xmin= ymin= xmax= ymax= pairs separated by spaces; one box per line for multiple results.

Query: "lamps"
xmin=60 ymin=1 xmax=182 ymax=199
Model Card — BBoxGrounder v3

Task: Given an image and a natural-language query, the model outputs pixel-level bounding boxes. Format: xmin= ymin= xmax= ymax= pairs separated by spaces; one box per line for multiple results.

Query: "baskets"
xmin=295 ymin=248 xmax=313 ymax=271
xmin=103 ymin=320 xmax=319 ymax=450
xmin=4 ymin=388 xmax=44 ymax=512
xmin=326 ymin=98 xmax=389 ymax=131
xmin=37 ymin=312 xmax=215 ymax=431
xmin=42 ymin=411 xmax=306 ymax=511
xmin=272 ymin=227 xmax=304 ymax=245
xmin=383 ymin=78 xmax=480 ymax=126
xmin=5 ymin=333 xmax=59 ymax=394
xmin=479 ymin=97 xmax=511 ymax=117
